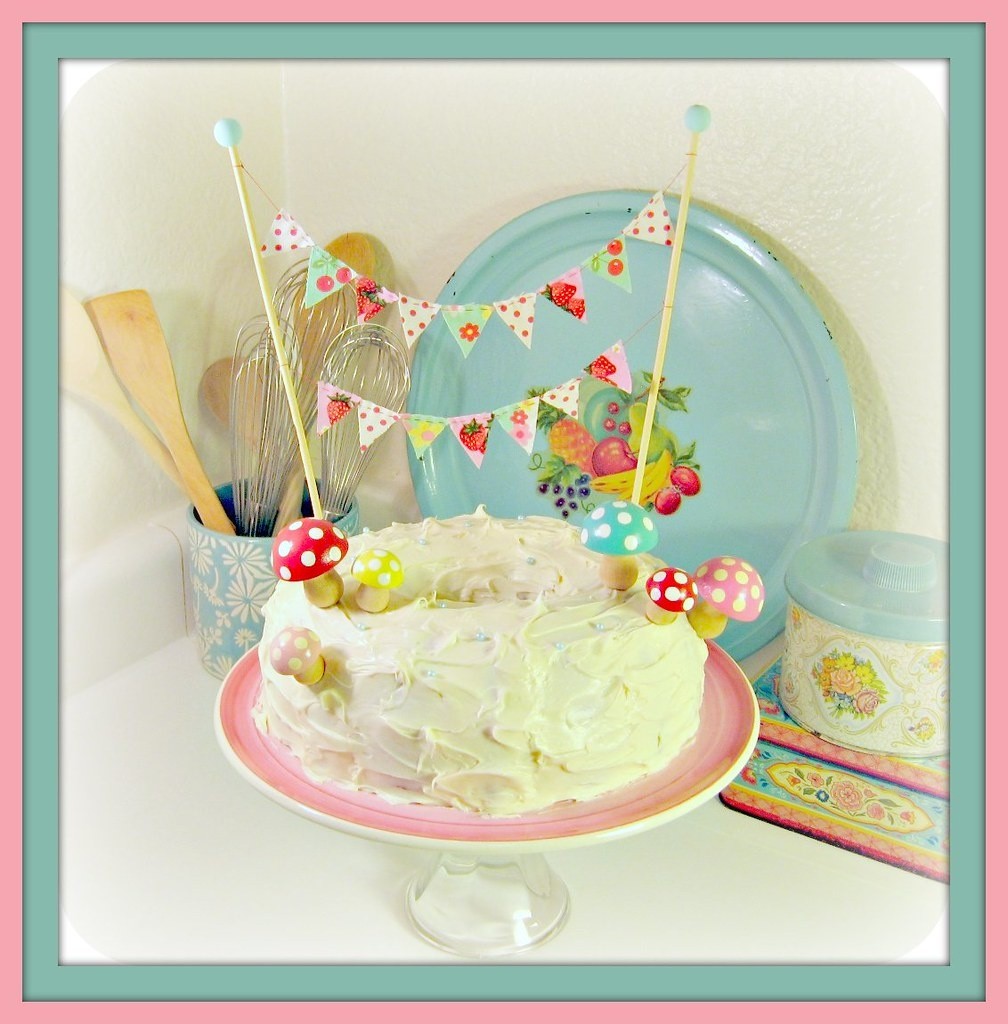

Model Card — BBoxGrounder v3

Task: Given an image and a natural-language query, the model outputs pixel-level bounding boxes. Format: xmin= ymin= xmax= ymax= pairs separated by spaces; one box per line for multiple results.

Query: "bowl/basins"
xmin=779 ymin=531 xmax=945 ymax=759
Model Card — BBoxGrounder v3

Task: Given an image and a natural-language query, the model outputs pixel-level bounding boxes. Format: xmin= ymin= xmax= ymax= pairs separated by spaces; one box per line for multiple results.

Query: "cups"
xmin=185 ymin=482 xmax=364 ymax=678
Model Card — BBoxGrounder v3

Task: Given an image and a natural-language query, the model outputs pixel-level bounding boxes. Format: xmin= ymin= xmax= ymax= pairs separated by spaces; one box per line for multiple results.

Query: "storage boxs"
xmin=720 ymin=647 xmax=949 ymax=901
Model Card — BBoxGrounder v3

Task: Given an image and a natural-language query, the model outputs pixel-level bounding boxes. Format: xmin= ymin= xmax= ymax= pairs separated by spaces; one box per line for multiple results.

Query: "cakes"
xmin=249 ymin=490 xmax=715 ymax=821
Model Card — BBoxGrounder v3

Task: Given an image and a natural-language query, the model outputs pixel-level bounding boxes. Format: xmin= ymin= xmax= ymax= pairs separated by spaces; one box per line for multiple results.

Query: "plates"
xmin=411 ymin=196 xmax=857 ymax=659
xmin=209 ymin=633 xmax=761 ymax=859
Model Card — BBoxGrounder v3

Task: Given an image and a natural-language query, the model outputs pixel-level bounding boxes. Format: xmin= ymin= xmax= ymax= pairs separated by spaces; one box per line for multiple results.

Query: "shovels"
xmin=59 ymin=288 xmax=197 ymax=507
xmin=82 ymin=288 xmax=239 ymax=535
